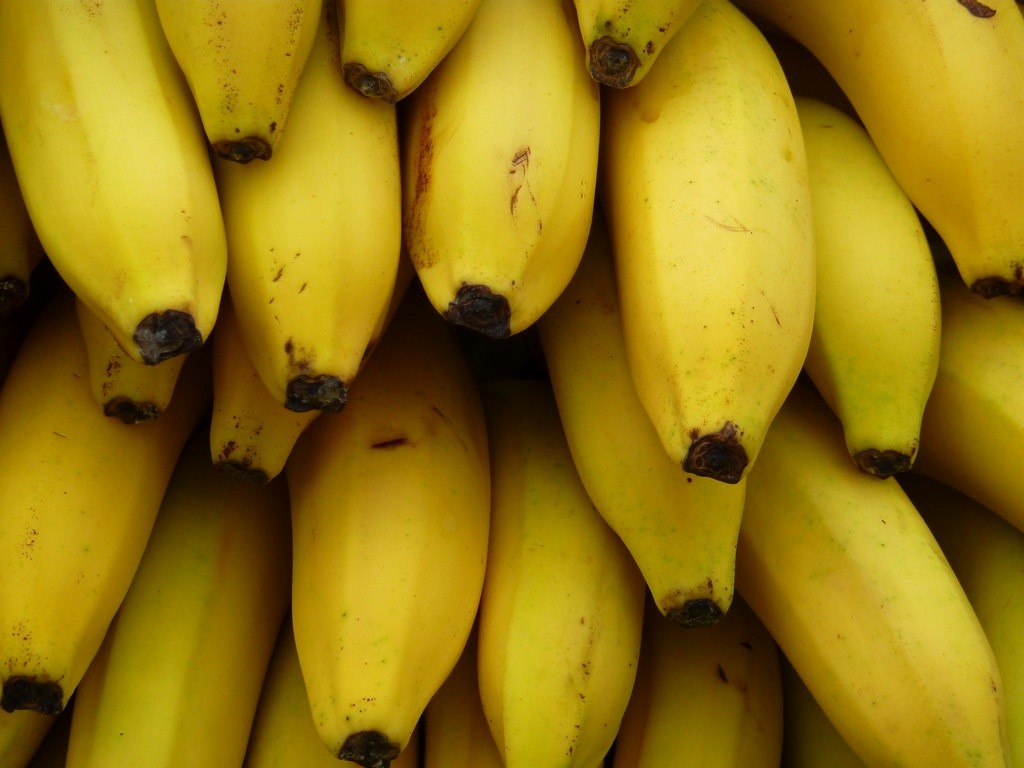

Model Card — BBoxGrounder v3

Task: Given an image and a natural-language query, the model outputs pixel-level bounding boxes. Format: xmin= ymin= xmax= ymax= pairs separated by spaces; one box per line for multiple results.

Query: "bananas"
xmin=0 ymin=0 xmax=1022 ymax=768
